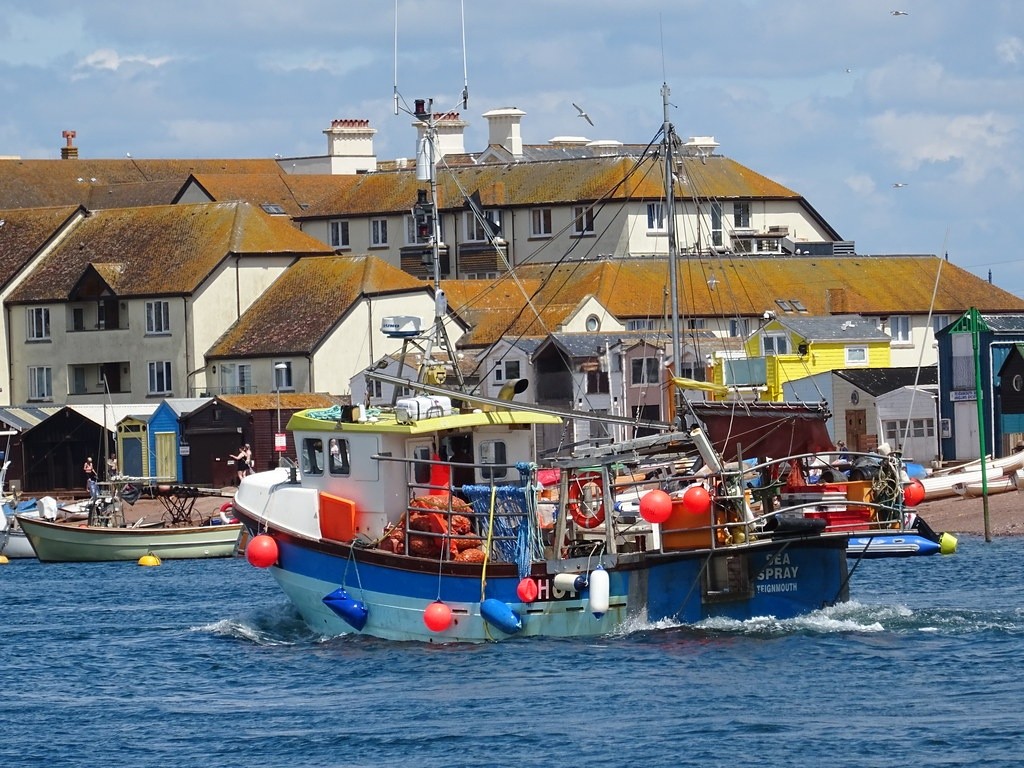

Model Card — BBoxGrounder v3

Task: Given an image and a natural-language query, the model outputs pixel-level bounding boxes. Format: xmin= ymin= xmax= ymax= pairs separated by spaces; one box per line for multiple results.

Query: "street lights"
xmin=274 ymin=364 xmax=287 ymax=466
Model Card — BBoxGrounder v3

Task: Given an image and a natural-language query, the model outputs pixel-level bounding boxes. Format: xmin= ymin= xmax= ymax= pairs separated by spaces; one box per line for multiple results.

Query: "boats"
xmin=1008 ymin=467 xmax=1024 ymax=489
xmin=915 ymin=466 xmax=1005 ymax=501
xmin=948 ymin=446 xmax=1024 ymax=474
xmin=950 ymin=474 xmax=1017 ymax=499
xmin=928 ymin=453 xmax=993 ymax=478
xmin=14 ymin=376 xmax=244 ymax=561
xmin=0 ymin=528 xmax=38 ymax=559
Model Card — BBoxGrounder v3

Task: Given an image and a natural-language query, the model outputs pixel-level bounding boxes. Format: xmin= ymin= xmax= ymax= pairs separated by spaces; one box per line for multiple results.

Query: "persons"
xmin=837 ymin=440 xmax=850 ymax=461
xmin=330 ymin=439 xmax=339 ymax=460
xmin=244 ymin=443 xmax=256 ymax=475
xmin=229 ymin=446 xmax=249 ymax=481
xmin=84 ymin=456 xmax=98 ymax=492
xmin=107 ymin=453 xmax=117 ymax=491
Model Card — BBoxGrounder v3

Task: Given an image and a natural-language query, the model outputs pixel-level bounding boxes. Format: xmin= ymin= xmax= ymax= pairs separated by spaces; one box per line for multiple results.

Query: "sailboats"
xmin=217 ymin=2 xmax=967 ymax=655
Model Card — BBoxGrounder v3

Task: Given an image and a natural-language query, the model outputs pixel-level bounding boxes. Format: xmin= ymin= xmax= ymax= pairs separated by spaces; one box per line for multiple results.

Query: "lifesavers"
xmin=568 ymin=471 xmax=613 ymax=529
xmin=772 ymin=459 xmax=800 ymax=502
xmin=219 ymin=502 xmax=239 ymax=524
